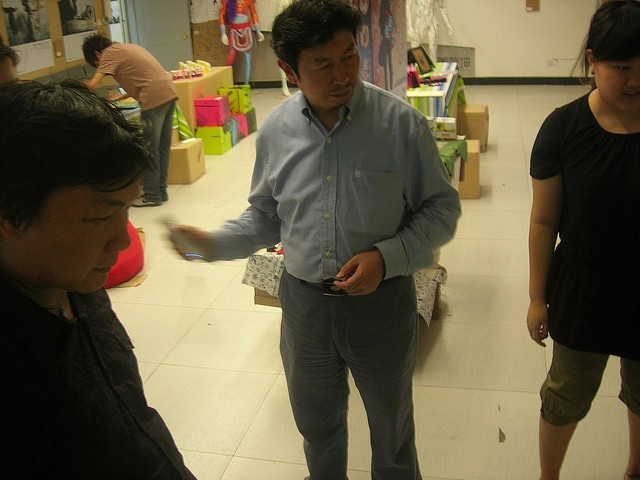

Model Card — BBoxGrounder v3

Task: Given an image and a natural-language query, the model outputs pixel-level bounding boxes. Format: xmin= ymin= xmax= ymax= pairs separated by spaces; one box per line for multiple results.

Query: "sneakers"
xmin=132 ymin=195 xmax=162 ymax=207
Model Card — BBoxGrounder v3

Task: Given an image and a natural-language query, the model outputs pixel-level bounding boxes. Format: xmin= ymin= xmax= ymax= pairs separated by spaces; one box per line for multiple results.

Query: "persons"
xmin=167 ymin=0 xmax=461 ymax=480
xmin=2 ymin=79 xmax=196 ymax=479
xmin=0 ymin=44 xmax=21 ymax=84
xmin=527 ymin=0 xmax=640 ymax=479
xmin=217 ymin=0 xmax=264 ymax=86
xmin=405 ymin=0 xmax=454 ymax=64
xmin=82 ymin=34 xmax=179 ymax=207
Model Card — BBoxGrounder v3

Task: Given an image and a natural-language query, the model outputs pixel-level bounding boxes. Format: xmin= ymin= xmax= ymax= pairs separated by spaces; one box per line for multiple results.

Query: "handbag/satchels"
xmin=407 ymin=62 xmax=420 ymax=88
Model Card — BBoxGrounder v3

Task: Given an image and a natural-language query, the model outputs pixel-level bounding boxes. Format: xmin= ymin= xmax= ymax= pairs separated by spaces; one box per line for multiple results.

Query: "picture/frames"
xmin=409 ymin=47 xmax=433 ymax=74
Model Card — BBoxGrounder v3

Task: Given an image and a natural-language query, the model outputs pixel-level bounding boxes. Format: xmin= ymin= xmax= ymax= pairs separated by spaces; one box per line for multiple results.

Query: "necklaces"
xmin=35 ymin=291 xmax=68 ymax=319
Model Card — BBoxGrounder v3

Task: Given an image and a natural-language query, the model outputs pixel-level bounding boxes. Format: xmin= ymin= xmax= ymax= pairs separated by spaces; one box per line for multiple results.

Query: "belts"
xmin=300 ymin=279 xmax=345 ymax=297
